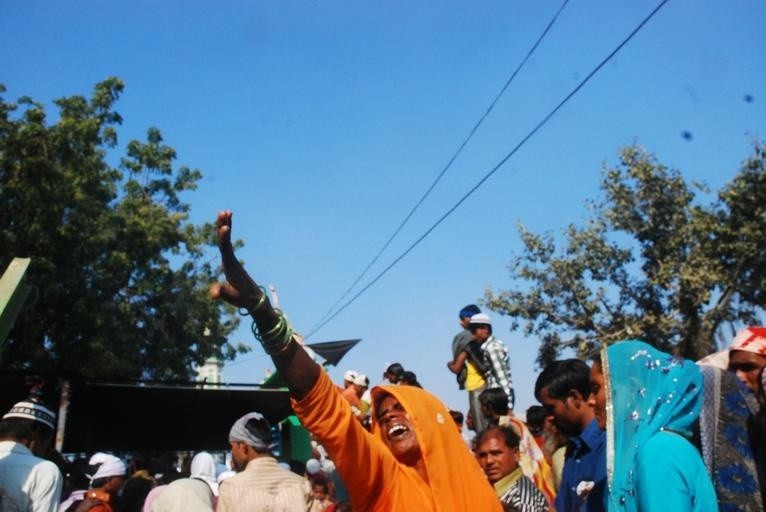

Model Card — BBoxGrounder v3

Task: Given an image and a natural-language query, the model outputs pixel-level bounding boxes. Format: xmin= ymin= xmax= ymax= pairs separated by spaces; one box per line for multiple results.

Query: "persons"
xmin=448 ymin=304 xmax=766 ymax=512
xmin=1 ymin=363 xmax=423 ymax=511
xmin=208 ymin=208 xmax=507 ymax=512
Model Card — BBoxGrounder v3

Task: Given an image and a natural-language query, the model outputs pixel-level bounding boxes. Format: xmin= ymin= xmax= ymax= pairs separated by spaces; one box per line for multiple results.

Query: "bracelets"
xmin=238 ymin=286 xmax=268 ymax=316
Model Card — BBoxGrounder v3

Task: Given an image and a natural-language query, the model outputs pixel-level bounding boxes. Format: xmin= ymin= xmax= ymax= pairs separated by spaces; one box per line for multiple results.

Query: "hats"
xmin=730 ymin=327 xmax=766 ymax=356
xmin=2 ymin=378 xmax=56 ymax=429
xmin=470 ymin=313 xmax=491 ymax=324
xmin=344 ymin=370 xmax=359 ymax=382
xmin=354 ymin=375 xmax=369 ymax=386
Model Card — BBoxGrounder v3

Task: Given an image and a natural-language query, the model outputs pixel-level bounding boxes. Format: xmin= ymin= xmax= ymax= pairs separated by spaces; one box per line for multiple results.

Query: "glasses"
xmin=730 ymin=362 xmax=765 ymax=372
